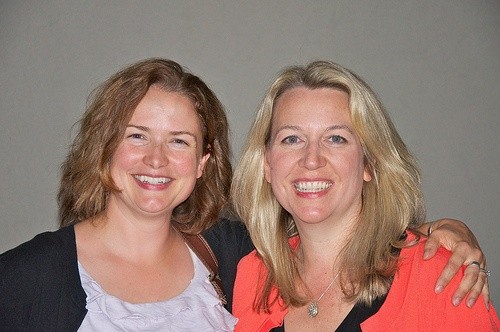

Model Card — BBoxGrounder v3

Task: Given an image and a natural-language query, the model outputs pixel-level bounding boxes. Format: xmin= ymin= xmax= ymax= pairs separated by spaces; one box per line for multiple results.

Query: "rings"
xmin=467 ymin=260 xmax=481 ymax=270
xmin=480 ymin=268 xmax=491 ymax=277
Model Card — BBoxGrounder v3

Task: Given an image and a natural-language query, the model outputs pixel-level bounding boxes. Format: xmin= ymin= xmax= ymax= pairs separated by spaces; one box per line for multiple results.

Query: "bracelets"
xmin=428 ymin=221 xmax=434 ymax=237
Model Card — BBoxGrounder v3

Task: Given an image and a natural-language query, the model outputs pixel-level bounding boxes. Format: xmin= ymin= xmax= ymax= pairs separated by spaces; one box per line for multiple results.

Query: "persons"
xmin=0 ymin=57 xmax=490 ymax=332
xmin=229 ymin=61 xmax=500 ymax=332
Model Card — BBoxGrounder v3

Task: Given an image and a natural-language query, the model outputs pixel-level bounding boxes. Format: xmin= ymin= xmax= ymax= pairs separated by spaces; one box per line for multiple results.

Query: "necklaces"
xmin=307 ymin=267 xmax=343 ymax=318
